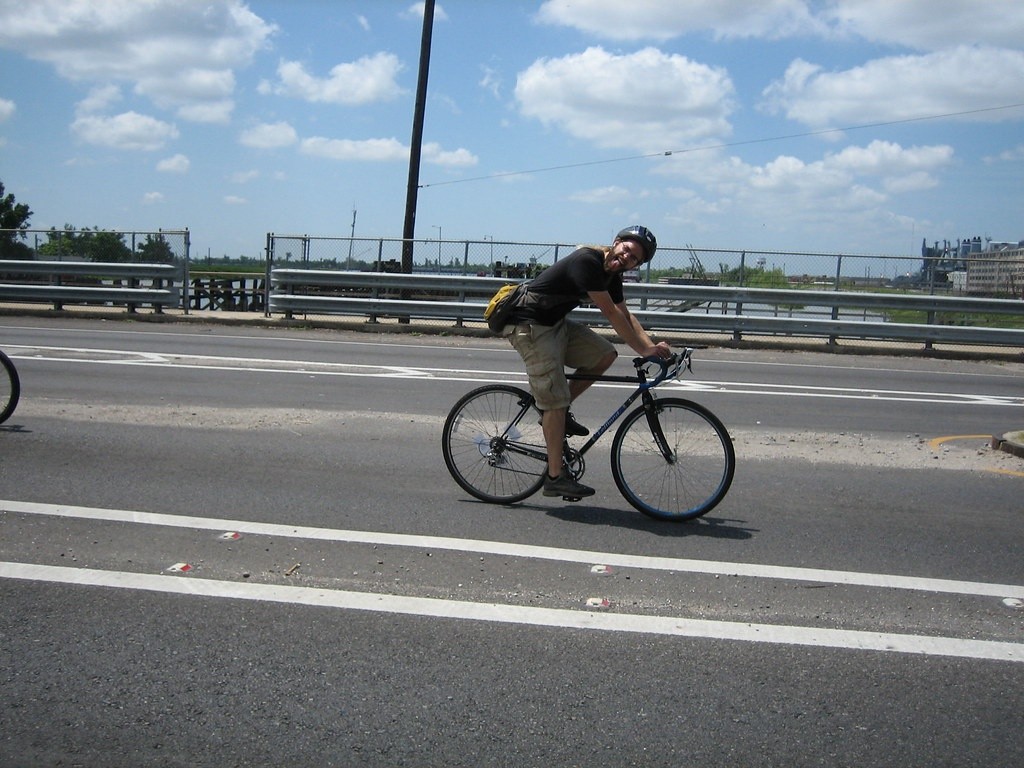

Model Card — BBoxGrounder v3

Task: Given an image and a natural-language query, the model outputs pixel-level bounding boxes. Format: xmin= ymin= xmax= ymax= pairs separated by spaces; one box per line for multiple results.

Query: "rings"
xmin=658 ymin=352 xmax=662 ymax=355
xmin=667 ymin=349 xmax=669 ymax=352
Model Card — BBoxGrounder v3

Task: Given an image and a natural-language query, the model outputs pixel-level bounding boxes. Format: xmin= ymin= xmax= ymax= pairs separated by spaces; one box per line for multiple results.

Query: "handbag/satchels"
xmin=484 ymin=285 xmax=525 ymax=333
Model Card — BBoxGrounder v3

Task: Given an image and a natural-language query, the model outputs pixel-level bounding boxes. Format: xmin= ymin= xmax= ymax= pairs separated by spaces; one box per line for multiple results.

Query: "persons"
xmin=507 ymin=225 xmax=674 ymax=496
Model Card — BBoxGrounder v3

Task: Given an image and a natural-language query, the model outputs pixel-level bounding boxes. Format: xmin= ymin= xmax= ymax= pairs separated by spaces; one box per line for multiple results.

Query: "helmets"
xmin=614 ymin=225 xmax=657 ymax=263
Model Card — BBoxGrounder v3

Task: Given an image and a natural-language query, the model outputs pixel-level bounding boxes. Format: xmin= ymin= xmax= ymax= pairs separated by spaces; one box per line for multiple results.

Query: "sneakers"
xmin=543 ymin=470 xmax=595 ymax=497
xmin=538 ymin=412 xmax=589 ymax=436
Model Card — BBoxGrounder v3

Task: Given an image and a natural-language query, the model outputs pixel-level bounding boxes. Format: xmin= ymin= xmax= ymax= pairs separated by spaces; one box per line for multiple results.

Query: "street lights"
xmin=431 ymin=224 xmax=443 ymax=276
xmin=483 ymin=234 xmax=493 ymax=277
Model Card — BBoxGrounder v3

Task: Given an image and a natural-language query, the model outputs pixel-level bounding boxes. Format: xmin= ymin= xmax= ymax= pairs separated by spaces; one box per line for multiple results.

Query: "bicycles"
xmin=439 ymin=343 xmax=735 ymax=523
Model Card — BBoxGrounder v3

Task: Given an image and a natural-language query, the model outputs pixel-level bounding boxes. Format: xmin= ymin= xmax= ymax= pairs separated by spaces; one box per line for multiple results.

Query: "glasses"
xmin=621 ymin=240 xmax=638 ymax=265
